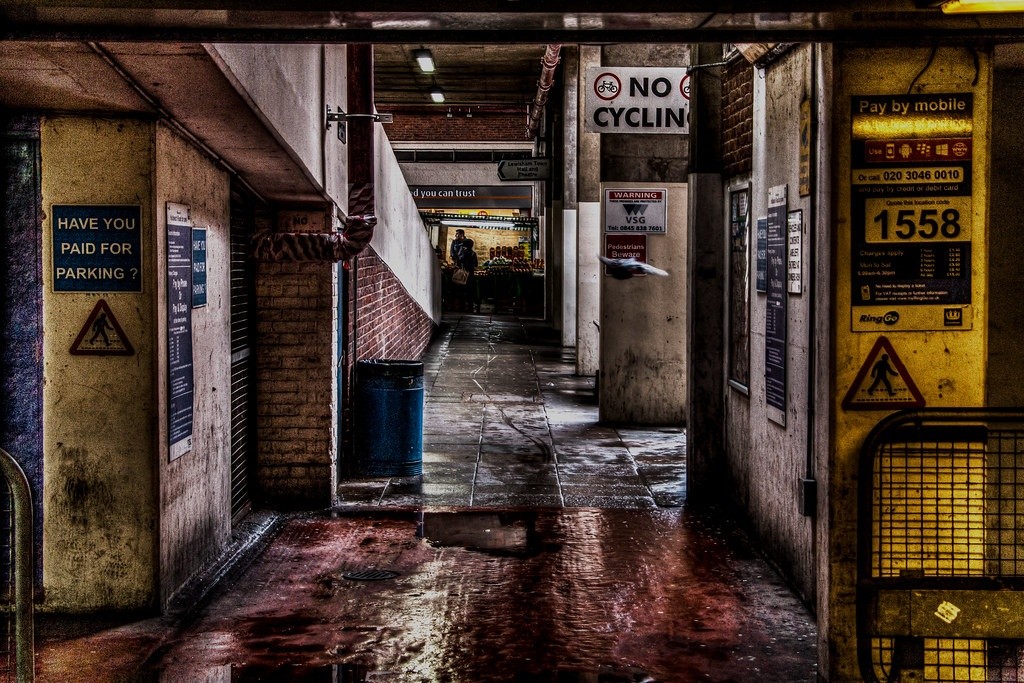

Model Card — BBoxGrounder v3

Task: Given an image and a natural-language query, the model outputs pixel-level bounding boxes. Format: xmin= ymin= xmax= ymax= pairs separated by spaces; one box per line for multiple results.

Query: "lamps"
xmin=411 ymin=48 xmax=436 ymax=72
xmin=428 ymin=87 xmax=445 ymax=102
xmin=938 ymin=0 xmax=1024 ymax=14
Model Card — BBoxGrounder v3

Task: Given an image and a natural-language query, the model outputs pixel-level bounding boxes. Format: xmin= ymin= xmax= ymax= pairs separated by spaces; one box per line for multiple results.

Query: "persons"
xmin=450 ymin=229 xmax=478 ymax=312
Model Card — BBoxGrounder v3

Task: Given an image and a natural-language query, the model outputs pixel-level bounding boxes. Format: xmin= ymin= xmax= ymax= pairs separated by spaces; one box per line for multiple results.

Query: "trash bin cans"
xmin=344 ymin=358 xmax=423 ymax=478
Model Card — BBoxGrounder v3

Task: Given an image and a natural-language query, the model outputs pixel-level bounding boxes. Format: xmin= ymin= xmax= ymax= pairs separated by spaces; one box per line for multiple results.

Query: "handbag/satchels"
xmin=453 ymin=262 xmax=470 ymax=284
